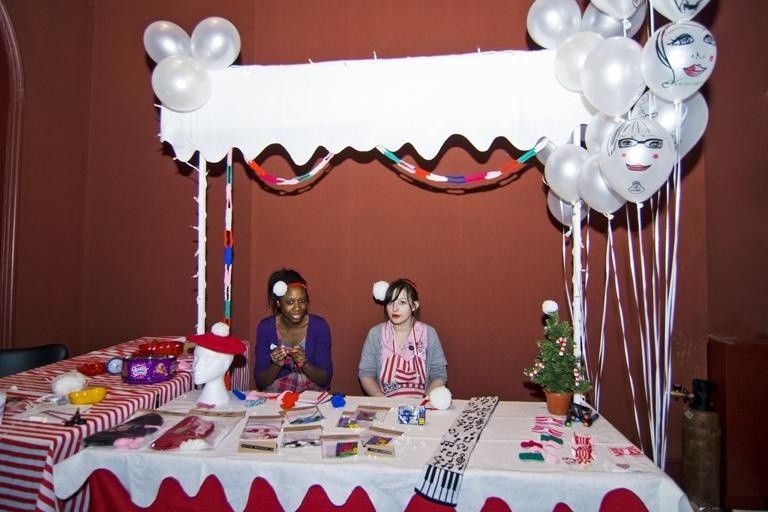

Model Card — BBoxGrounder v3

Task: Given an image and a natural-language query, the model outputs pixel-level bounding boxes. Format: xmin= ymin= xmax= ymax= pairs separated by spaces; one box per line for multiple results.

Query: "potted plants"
xmin=522 ymin=319 xmax=593 ymax=414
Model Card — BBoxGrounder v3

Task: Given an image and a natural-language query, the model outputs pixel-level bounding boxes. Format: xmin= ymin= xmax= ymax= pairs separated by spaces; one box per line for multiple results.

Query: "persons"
xmin=185 ymin=323 xmax=245 ymax=404
xmin=358 ymin=278 xmax=448 ymax=399
xmin=255 ymin=268 xmax=333 ymax=397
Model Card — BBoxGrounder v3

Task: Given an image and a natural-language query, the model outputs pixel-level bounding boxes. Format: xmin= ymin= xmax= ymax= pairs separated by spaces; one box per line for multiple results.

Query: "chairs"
xmin=0 ymin=344 xmax=66 ymax=380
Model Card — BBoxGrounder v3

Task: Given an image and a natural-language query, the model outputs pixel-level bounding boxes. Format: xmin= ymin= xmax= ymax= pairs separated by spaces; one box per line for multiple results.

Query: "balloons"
xmin=527 ymin=1 xmax=716 ymax=224
xmin=143 ymin=16 xmax=242 ymax=112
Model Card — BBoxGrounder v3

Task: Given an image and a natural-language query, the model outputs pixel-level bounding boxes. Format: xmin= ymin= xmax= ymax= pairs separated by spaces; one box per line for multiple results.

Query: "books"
xmin=296 ymin=389 xmax=329 ymax=403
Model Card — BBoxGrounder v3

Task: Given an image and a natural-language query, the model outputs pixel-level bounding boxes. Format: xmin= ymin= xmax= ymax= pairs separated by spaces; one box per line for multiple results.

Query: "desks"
xmin=0 ymin=334 xmax=249 ymax=512
xmin=53 ymin=390 xmax=700 ymax=512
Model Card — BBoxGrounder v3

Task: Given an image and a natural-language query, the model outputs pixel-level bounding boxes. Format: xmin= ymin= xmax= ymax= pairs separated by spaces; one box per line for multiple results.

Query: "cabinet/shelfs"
xmin=705 ymin=336 xmax=767 ymax=510
